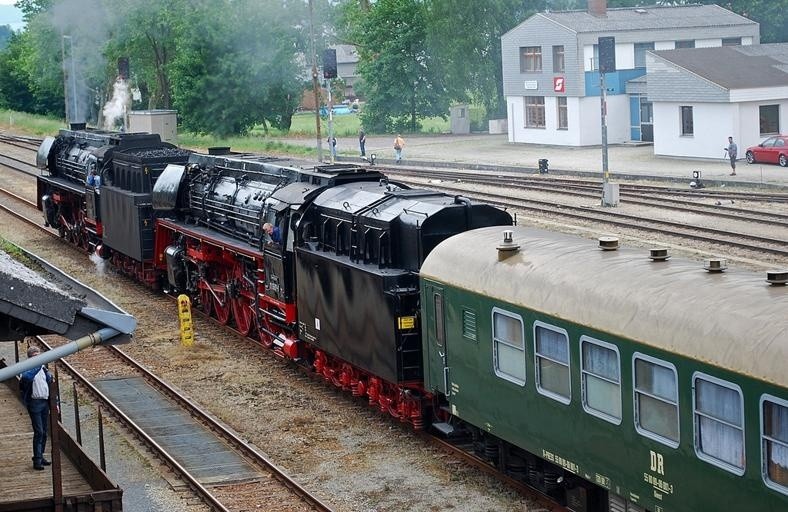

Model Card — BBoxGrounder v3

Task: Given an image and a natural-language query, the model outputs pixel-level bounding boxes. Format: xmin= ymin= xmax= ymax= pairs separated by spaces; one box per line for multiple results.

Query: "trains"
xmin=32 ymin=121 xmax=787 ymax=512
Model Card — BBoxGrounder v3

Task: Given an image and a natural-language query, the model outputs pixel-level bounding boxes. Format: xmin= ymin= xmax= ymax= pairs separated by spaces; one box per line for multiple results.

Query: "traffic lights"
xmin=117 ymin=56 xmax=129 ymax=80
xmin=598 ymin=35 xmax=614 ymax=73
xmin=323 ymin=48 xmax=337 ymax=77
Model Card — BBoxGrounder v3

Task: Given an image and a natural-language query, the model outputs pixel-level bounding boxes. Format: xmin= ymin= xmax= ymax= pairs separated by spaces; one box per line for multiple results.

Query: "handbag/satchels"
xmin=395 ymin=143 xmax=400 ymax=150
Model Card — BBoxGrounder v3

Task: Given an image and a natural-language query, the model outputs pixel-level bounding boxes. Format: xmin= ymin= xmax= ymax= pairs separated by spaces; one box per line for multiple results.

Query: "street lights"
xmin=62 ymin=33 xmax=78 ymax=122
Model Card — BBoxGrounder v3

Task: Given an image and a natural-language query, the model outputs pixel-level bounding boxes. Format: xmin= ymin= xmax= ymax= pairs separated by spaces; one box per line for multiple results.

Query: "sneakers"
xmin=32 ymin=457 xmax=51 ymax=470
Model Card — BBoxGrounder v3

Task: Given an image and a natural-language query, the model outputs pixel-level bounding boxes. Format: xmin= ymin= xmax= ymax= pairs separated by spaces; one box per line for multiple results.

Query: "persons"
xmin=263 ymin=223 xmax=282 ymax=245
xmin=327 ymin=136 xmax=336 ymax=156
xmin=393 ymin=135 xmax=404 ymax=164
xmin=724 ymin=137 xmax=737 ymax=175
xmin=91 ymin=169 xmax=100 ymax=195
xmin=359 ymin=128 xmax=365 ymax=156
xmin=83 ymin=169 xmax=95 ymax=186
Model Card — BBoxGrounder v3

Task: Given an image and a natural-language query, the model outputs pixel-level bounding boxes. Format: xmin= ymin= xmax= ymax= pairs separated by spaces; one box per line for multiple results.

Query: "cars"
xmin=744 ymin=134 xmax=787 ymax=166
